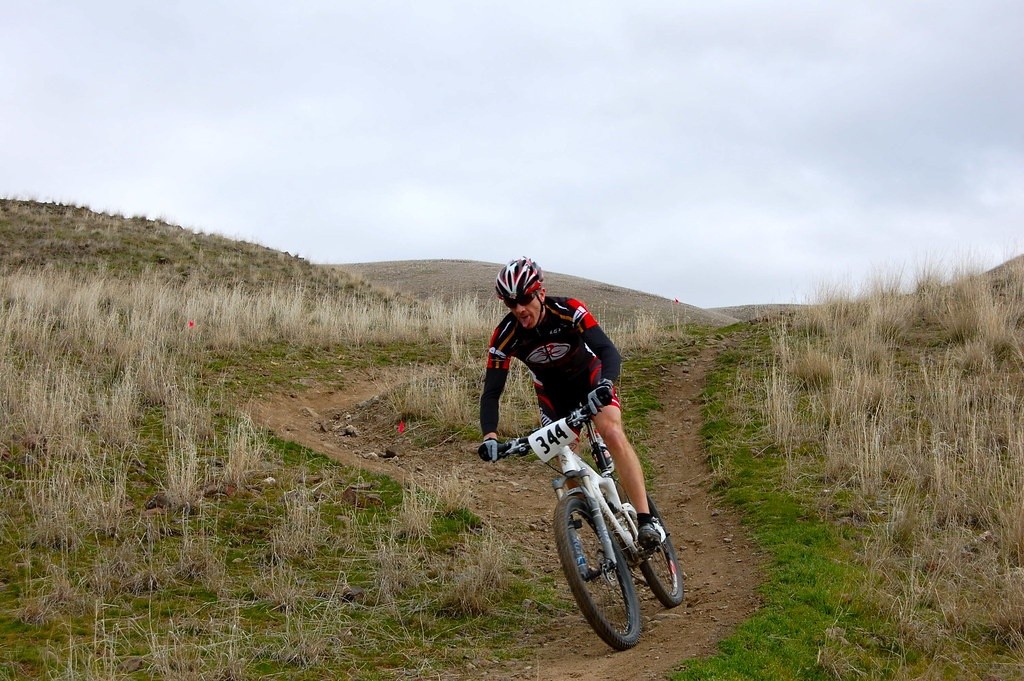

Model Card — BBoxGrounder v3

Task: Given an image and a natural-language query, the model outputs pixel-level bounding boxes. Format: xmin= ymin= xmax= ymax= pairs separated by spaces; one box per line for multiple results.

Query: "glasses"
xmin=503 ymin=286 xmax=542 ymax=308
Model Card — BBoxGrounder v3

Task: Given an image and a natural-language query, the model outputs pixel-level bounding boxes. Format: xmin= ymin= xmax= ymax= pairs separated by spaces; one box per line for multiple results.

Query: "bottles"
xmin=586 ymin=429 xmax=614 ymax=478
xmin=607 ymin=502 xmax=634 ymax=542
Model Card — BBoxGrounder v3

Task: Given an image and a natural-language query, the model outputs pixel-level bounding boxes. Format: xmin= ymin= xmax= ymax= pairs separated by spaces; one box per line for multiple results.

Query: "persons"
xmin=478 ymin=257 xmax=663 ymax=548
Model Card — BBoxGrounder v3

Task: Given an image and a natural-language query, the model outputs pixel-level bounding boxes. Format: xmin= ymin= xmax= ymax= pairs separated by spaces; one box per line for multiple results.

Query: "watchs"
xmin=597 ymin=378 xmax=613 ymax=387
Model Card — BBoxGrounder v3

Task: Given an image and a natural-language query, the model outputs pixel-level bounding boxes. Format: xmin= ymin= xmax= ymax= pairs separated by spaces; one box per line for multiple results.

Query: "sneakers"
xmin=637 ymin=516 xmax=667 ymax=548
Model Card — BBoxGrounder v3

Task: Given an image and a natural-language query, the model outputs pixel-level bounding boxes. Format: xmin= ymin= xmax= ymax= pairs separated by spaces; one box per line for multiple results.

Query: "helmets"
xmin=495 ymin=256 xmax=545 ymax=299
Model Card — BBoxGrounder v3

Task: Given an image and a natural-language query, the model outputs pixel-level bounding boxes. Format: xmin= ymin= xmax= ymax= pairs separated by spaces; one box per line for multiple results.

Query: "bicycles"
xmin=490 ymin=388 xmax=684 ymax=649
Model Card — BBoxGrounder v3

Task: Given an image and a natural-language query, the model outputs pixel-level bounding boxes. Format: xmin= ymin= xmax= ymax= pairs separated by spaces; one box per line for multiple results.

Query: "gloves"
xmin=477 ymin=440 xmax=499 ymax=463
xmin=586 ymin=382 xmax=614 ymax=415
